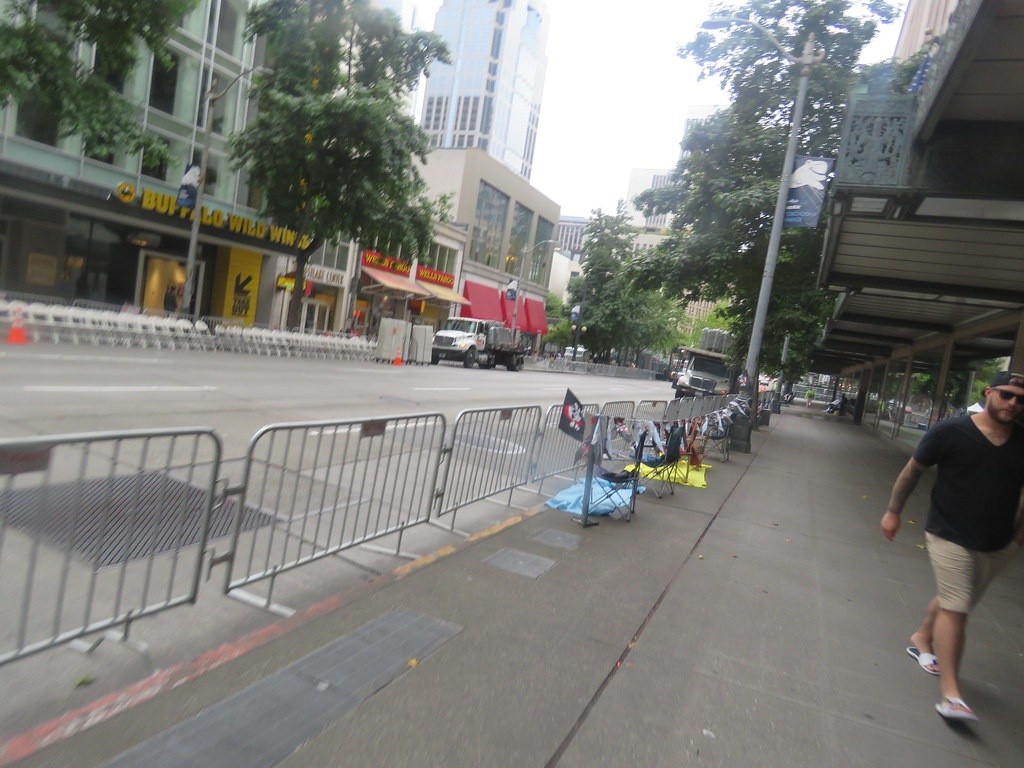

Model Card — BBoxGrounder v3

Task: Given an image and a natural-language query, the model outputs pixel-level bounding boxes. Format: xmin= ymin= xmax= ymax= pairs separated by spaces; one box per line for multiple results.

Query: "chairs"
xmin=588 ymin=413 xmax=733 ymax=522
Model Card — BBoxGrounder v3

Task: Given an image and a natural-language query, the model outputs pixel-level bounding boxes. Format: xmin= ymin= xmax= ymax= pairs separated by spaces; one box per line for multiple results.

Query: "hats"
xmin=981 ymin=370 xmax=1024 ymax=397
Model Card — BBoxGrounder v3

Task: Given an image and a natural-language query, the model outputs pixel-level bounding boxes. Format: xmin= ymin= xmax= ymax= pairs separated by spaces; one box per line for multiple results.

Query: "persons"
xmin=880 ymin=371 xmax=1024 ymax=720
xmin=804 ymin=388 xmax=815 ymax=408
xmin=825 ymin=393 xmax=844 ymax=413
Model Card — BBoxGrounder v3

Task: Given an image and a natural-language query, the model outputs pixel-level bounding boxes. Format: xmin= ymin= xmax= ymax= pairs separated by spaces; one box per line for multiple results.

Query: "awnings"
xmin=359 ymin=267 xmax=473 ymax=307
xmin=524 ymin=298 xmax=547 ymax=334
xmin=460 ymin=280 xmax=503 ymax=323
xmin=500 ymin=291 xmax=528 ymax=331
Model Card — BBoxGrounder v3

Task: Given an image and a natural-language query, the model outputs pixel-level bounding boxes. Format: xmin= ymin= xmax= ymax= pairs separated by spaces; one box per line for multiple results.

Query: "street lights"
xmin=512 ymin=240 xmax=558 ymax=335
xmin=572 ymin=272 xmax=614 ymax=360
xmin=702 ymin=15 xmax=815 ymax=453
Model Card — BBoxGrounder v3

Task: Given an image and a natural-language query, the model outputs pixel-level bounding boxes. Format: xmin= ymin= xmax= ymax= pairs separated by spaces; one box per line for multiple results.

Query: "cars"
xmin=758 ymin=374 xmax=779 ymax=386
xmin=671 ymin=359 xmax=689 ymax=388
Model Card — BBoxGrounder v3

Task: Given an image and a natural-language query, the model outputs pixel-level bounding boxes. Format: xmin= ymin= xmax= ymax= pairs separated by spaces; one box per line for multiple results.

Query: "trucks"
xmin=432 ymin=316 xmax=531 ymax=371
xmin=674 ymin=356 xmax=740 ymax=399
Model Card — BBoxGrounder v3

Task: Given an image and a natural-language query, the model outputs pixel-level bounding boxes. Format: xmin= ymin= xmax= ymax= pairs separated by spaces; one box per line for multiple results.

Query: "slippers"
xmin=936 ymin=698 xmax=979 ymax=722
xmin=907 ymin=646 xmax=941 ymax=675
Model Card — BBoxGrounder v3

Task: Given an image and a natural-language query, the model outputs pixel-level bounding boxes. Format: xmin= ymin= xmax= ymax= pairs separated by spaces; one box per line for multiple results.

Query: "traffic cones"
xmin=393 ymin=346 xmax=403 ymax=365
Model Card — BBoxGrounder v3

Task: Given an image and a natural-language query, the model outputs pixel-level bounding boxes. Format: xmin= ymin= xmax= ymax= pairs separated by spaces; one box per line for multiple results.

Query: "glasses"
xmin=990 ymin=386 xmax=1024 ymax=405
